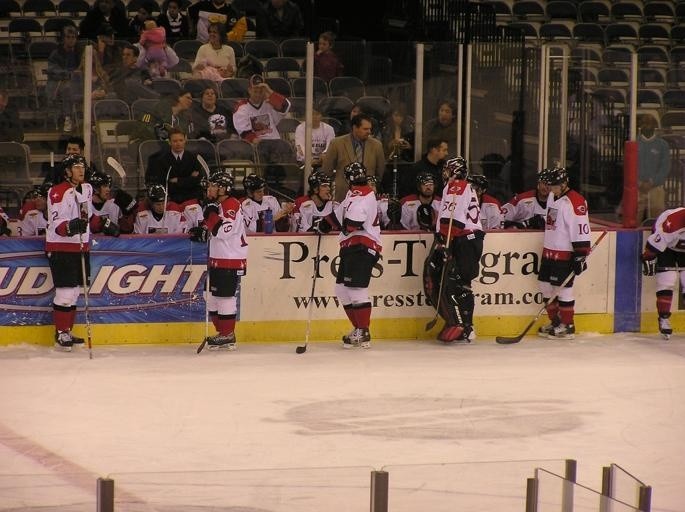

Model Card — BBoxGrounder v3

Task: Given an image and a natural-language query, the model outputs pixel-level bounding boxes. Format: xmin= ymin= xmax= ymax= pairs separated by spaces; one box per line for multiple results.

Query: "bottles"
xmin=263 ymin=207 xmax=274 ymax=234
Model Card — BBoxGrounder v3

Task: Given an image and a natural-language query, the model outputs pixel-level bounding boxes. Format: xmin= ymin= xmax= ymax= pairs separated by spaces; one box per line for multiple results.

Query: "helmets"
xmin=415 ymin=156 xmax=488 ymax=195
xmin=309 ymin=162 xmax=376 ymax=189
xmin=209 ymin=172 xmax=266 ymax=192
xmin=35 ymin=153 xmax=112 ymax=197
xmin=537 ymin=168 xmax=569 ymax=185
xmin=147 ymin=184 xmax=166 ymax=202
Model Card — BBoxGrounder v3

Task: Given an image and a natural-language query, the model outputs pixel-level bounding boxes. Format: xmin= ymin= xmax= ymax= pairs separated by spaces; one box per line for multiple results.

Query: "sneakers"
xmin=538 ymin=319 xmax=574 ymax=336
xmin=343 ymin=328 xmax=370 ymax=344
xmin=208 ymin=332 xmax=235 ymax=344
xmin=55 ymin=330 xmax=84 ymax=346
xmin=658 ymin=312 xmax=672 ymax=334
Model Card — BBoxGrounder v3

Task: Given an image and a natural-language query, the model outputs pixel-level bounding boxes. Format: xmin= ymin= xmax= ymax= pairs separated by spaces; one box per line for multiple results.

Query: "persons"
xmin=299 ymin=31 xmax=343 ymax=83
xmin=636 ymin=115 xmax=672 ymax=227
xmin=315 ymin=161 xmax=384 ymax=352
xmin=536 ymin=165 xmax=594 ymax=341
xmin=189 ymin=172 xmax=250 ymax=352
xmin=294 ymin=98 xmax=457 ymax=169
xmin=423 ymin=153 xmax=486 ymax=344
xmin=151 ymin=129 xmax=200 ymax=204
xmin=640 ymin=206 xmax=685 ymax=341
xmin=256 ymin=1 xmax=305 ymax=40
xmin=1 ymin=139 xmax=547 ymax=237
xmin=43 ymin=153 xmax=119 ymax=353
xmin=320 ymin=115 xmax=384 ymax=203
xmin=48 ymin=1 xmax=291 ymax=147
xmin=45 ymin=135 xmax=96 ymax=185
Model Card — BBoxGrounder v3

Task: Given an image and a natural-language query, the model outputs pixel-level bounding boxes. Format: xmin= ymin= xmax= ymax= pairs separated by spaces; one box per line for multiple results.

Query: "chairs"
xmin=2 ymin=2 xmax=685 ymax=235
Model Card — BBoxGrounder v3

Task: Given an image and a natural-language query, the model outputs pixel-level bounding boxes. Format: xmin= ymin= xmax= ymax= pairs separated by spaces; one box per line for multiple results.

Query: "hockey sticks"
xmin=296 ymin=233 xmax=321 ymax=353
xmin=107 ymin=157 xmax=127 ymax=192
xmin=161 ymin=165 xmax=172 ymax=231
xmin=495 ymin=228 xmax=607 ymax=344
xmin=425 ymin=184 xmax=457 ymax=332
xmin=196 ymin=155 xmax=210 ymax=181
xmin=196 ymin=230 xmax=212 ymax=353
xmin=75 ymin=194 xmax=93 ymax=359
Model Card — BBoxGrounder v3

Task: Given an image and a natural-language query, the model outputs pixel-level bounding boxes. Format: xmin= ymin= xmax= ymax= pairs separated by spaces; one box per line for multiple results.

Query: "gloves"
xmin=101 ymin=219 xmax=120 ymax=237
xmin=202 ymin=200 xmax=218 ymax=216
xmin=115 ymin=191 xmax=138 ymax=213
xmin=570 ymin=254 xmax=587 ymax=275
xmin=189 ymin=227 xmax=207 ymax=242
xmin=313 ymin=217 xmax=331 ymax=234
xmin=66 ymin=218 xmax=87 ymax=237
xmin=641 ymin=255 xmax=657 ymax=276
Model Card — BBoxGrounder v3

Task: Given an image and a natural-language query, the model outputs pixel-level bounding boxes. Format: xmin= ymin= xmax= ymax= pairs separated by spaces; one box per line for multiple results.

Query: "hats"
xmin=249 ymin=74 xmax=264 ymax=88
